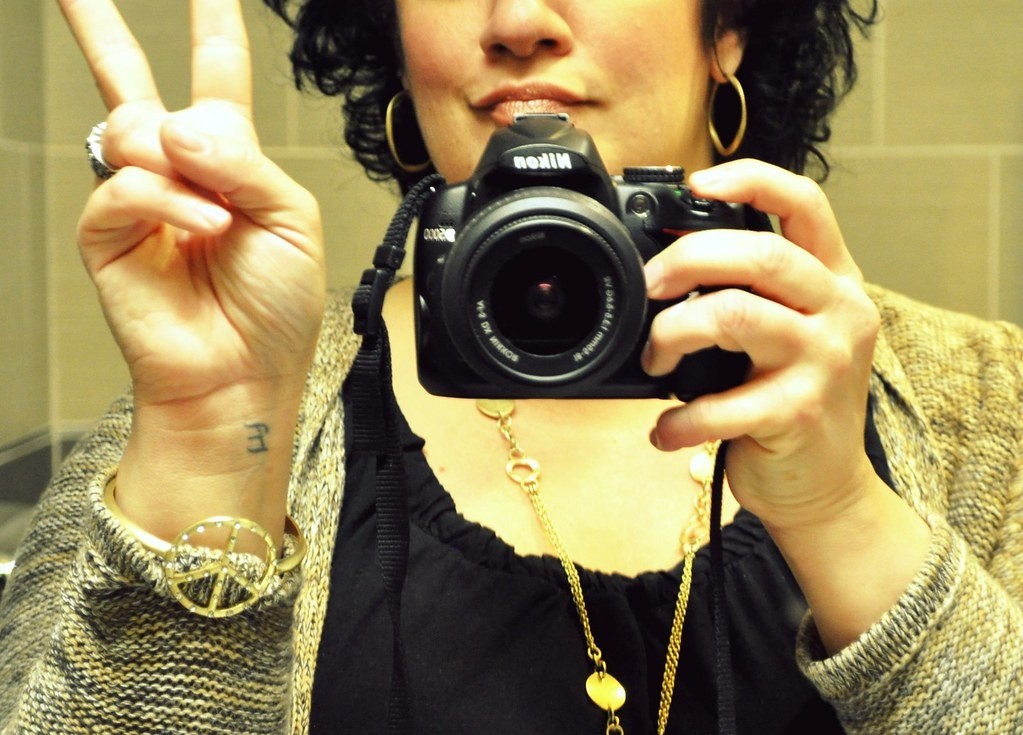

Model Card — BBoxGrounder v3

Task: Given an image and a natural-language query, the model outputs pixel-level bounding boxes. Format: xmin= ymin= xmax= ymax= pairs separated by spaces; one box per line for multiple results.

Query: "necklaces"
xmin=474 ymin=400 xmax=721 ymax=734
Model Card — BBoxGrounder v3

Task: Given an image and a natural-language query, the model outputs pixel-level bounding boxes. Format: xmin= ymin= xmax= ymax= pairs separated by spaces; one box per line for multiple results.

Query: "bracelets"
xmin=105 ymin=478 xmax=309 ymax=618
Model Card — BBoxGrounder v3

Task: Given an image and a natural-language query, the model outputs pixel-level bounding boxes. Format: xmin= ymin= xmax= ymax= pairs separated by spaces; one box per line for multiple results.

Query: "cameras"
xmin=414 ymin=111 xmax=776 ymax=404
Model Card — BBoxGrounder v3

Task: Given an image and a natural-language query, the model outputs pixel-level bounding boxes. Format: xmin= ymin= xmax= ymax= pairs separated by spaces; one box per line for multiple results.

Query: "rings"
xmin=86 ymin=121 xmax=119 ymax=179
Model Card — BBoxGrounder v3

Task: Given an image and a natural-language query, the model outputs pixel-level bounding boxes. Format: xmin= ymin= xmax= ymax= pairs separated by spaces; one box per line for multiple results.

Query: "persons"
xmin=0 ymin=0 xmax=1023 ymax=735
